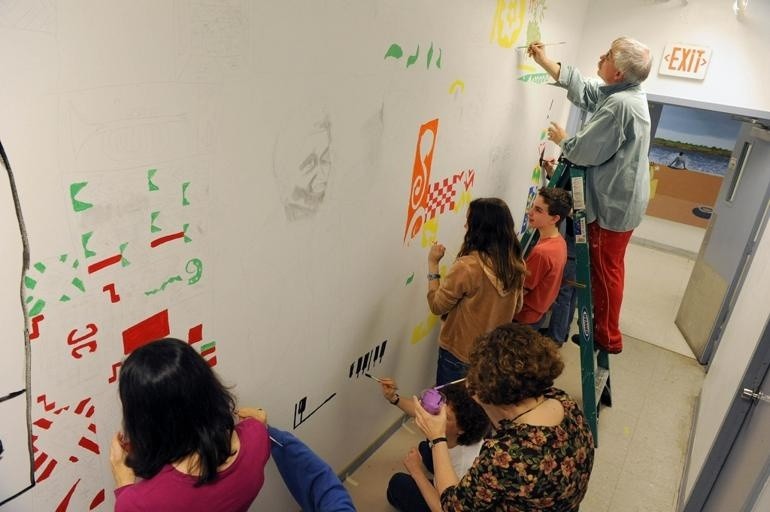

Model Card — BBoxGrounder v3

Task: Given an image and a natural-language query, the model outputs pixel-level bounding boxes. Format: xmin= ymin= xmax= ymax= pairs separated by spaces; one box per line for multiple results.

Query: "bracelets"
xmin=390 ymin=393 xmax=399 ymax=405
xmin=427 ymin=274 xmax=440 ymax=279
xmin=429 ymin=437 xmax=448 ymax=448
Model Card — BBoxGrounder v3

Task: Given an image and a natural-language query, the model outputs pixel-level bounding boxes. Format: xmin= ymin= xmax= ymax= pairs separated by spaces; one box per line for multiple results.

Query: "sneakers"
xmin=572 ymin=333 xmax=622 ymax=354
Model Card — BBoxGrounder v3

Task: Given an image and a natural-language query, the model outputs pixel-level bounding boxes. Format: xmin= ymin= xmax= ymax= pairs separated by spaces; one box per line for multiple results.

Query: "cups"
xmin=420 ymin=388 xmax=448 ymax=415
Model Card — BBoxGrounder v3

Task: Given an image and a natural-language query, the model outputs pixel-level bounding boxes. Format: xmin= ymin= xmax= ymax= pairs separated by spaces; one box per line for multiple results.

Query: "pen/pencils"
xmin=230 ymin=405 xmax=286 ymax=450
xmin=517 ymin=41 xmax=568 ymax=49
xmin=542 ymin=159 xmax=559 ymax=166
xmin=431 ymin=240 xmax=438 ymax=244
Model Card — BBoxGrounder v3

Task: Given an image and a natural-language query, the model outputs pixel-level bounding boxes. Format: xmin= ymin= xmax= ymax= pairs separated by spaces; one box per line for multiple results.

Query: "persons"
xmin=517 ymin=185 xmax=572 ymax=330
xmin=236 ymin=408 xmax=358 ymax=511
xmin=414 ymin=322 xmax=595 ymax=512
xmin=422 ymin=198 xmax=525 ymax=386
xmin=110 ymin=338 xmax=270 ymax=512
xmin=527 ymin=35 xmax=652 ymax=353
xmin=378 ymin=378 xmax=484 ymax=512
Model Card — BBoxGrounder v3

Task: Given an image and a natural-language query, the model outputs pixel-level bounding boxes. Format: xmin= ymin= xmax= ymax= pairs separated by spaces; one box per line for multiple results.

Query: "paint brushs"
xmin=365 ymin=373 xmax=400 ymax=391
xmin=432 ymin=378 xmax=468 ymax=390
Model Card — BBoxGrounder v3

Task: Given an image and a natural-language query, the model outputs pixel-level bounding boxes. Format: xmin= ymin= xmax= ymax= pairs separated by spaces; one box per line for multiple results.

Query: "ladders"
xmin=519 ymin=157 xmax=611 ymax=448
